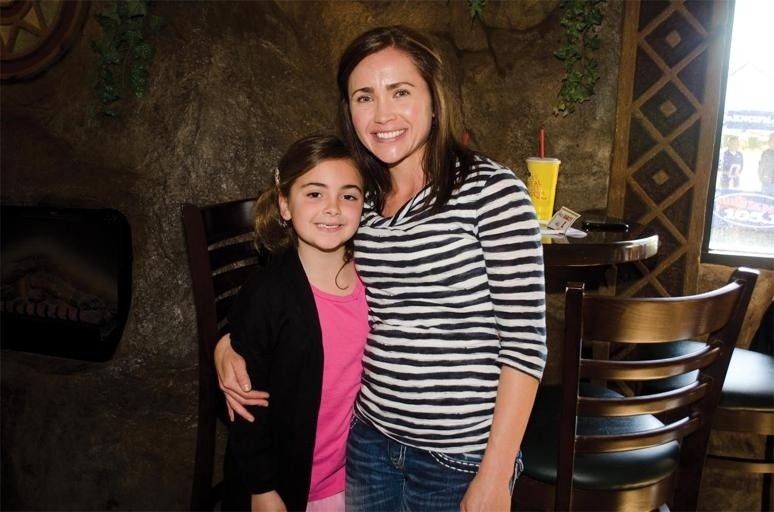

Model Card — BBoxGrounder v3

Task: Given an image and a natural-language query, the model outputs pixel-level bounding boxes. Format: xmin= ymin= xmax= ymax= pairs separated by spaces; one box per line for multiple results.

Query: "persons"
xmin=222 ymin=131 xmax=370 ymax=511
xmin=760 ymin=132 xmax=774 ymax=195
xmin=721 ymin=136 xmax=743 ymax=188
xmin=213 ymin=22 xmax=548 ymax=512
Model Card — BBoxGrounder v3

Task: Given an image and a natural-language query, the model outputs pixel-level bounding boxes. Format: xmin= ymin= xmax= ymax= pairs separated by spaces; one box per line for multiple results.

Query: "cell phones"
xmin=582 ymin=216 xmax=629 ymax=229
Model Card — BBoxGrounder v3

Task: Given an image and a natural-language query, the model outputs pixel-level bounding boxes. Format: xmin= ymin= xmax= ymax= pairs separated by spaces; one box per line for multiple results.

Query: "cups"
xmin=523 ymin=155 xmax=564 ymax=224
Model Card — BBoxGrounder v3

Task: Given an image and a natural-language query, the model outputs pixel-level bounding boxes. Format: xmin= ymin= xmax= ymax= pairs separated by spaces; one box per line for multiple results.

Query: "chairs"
xmin=519 ymin=267 xmax=772 ymax=512
xmin=180 ymin=185 xmax=258 ymax=512
